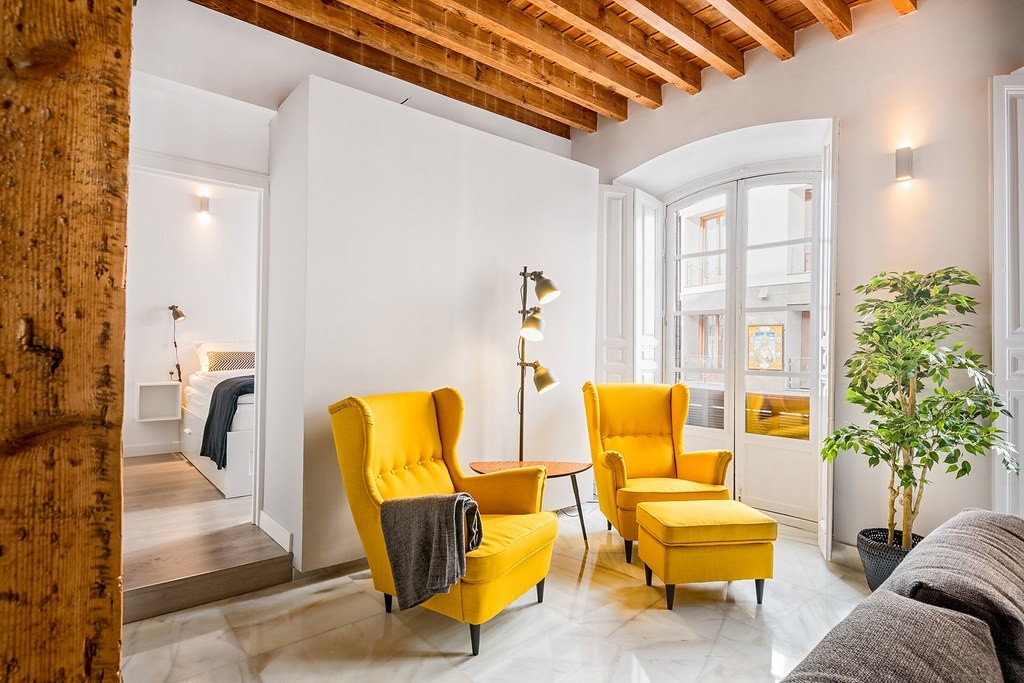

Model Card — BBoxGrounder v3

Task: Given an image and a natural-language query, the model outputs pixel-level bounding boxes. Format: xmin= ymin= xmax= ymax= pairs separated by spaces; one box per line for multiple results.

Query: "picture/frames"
xmin=747 ymin=322 xmax=786 ymax=372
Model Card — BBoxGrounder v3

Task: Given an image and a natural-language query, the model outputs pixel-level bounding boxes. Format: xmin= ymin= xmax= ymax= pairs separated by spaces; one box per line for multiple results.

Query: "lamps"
xmin=895 ymin=147 xmax=914 ymax=182
xmin=199 ymin=196 xmax=210 ymax=213
xmin=168 ymin=305 xmax=186 ymax=383
xmin=515 ymin=265 xmax=561 ymax=461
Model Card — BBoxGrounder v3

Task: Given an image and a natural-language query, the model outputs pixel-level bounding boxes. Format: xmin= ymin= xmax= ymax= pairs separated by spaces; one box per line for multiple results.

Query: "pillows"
xmin=193 ymin=341 xmax=257 ymax=373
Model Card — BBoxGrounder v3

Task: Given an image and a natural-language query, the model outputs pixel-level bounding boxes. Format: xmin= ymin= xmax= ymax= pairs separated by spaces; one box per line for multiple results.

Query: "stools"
xmin=635 ymin=499 xmax=778 ymax=612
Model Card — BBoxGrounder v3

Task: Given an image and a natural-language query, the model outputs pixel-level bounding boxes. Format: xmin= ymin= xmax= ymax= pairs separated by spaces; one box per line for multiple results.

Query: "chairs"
xmin=581 ymin=380 xmax=732 ymax=564
xmin=327 ymin=385 xmax=560 ymax=656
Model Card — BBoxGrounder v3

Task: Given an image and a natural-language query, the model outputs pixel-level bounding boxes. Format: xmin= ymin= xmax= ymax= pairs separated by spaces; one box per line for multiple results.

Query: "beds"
xmin=179 ymin=342 xmax=256 ymax=499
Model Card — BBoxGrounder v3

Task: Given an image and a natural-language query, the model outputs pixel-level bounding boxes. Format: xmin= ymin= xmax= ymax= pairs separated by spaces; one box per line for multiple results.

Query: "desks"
xmin=469 ymin=461 xmax=596 ymax=548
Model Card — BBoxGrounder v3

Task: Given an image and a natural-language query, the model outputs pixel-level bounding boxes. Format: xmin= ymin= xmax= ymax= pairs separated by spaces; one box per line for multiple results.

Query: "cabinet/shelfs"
xmin=134 ymin=380 xmax=183 ymax=422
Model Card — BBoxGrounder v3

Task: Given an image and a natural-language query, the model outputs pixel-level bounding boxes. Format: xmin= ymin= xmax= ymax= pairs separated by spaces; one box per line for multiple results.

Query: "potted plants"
xmin=820 ymin=265 xmax=1021 ymax=595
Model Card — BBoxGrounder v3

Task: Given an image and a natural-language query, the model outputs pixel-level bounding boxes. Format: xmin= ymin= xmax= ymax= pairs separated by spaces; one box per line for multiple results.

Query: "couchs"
xmin=780 ymin=508 xmax=1024 ymax=683
